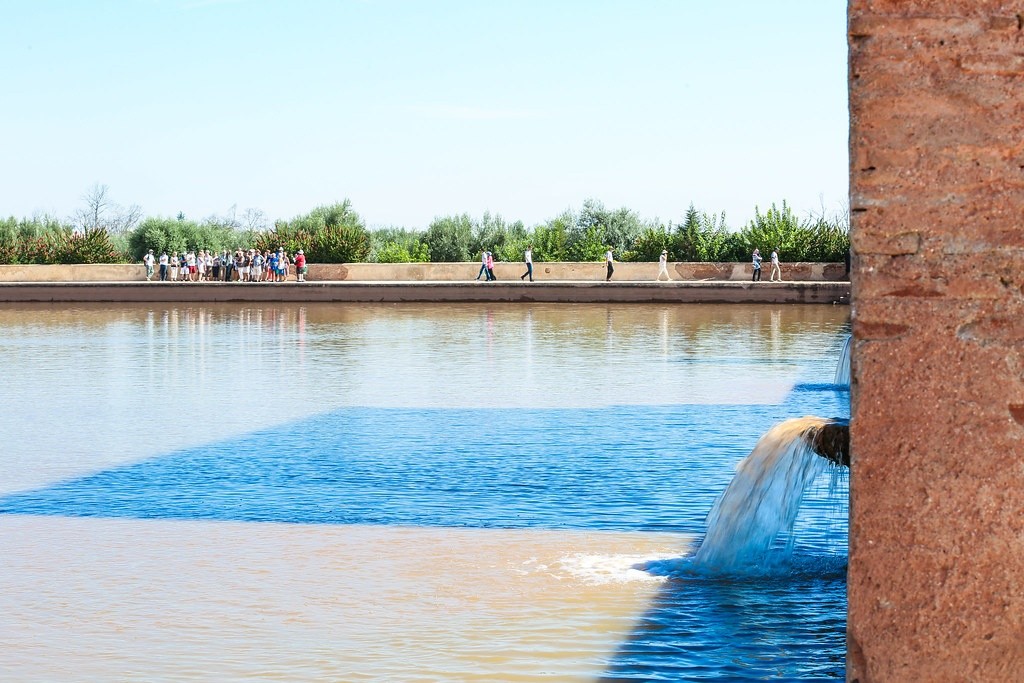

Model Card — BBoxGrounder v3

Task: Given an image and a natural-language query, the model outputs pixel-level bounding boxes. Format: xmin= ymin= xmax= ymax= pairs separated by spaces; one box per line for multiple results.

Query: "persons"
xmin=656 ymin=250 xmax=674 ymax=281
xmin=486 ymin=251 xmax=497 ymax=280
xmin=606 ymin=245 xmax=615 ymax=281
xmin=770 ymin=246 xmax=782 ymax=282
xmin=139 ymin=245 xmax=307 ymax=283
xmin=475 ymin=247 xmax=491 ymax=282
xmin=750 ymin=248 xmax=763 ymax=282
xmin=520 ymin=244 xmax=536 ymax=282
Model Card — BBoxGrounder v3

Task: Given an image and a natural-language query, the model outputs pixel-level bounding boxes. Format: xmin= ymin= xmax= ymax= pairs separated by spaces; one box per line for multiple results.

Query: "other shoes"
xmin=608 ymin=280 xmax=612 ymax=281
xmin=474 ymin=278 xmax=496 ymax=281
xmin=521 ymin=277 xmax=524 ymax=281
xmin=656 ymin=279 xmax=661 ymax=282
xmin=146 ymin=276 xmax=307 ymax=282
xmin=530 ymin=280 xmax=534 ymax=281
xmin=606 ymin=279 xmax=608 ymax=281
xmin=752 ymin=279 xmax=783 ymax=282
xmin=668 ymin=279 xmax=674 ymax=281
